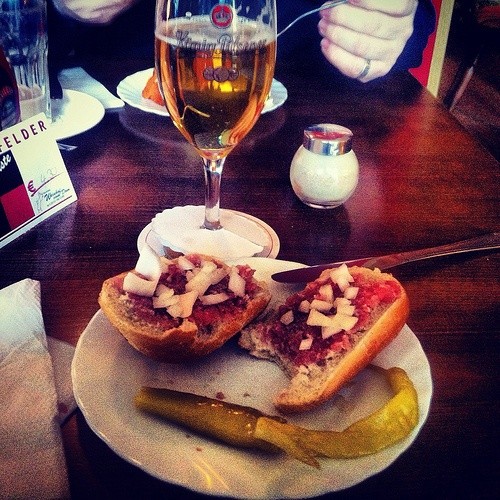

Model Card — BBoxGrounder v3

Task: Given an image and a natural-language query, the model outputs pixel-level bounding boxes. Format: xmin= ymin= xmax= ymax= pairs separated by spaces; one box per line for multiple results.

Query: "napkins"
xmin=1 ymin=274 xmax=85 ymax=500
xmin=57 ymin=65 xmax=125 ymax=109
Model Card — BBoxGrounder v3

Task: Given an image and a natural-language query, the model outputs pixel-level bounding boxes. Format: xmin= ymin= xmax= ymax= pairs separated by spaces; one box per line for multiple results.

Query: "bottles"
xmin=290 ymin=123 xmax=360 ymax=209
xmin=0 ymin=40 xmax=21 ymax=132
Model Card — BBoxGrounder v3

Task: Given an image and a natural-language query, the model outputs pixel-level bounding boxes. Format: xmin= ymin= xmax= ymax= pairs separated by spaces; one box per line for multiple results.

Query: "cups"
xmin=0 ymin=0 xmax=53 ymax=124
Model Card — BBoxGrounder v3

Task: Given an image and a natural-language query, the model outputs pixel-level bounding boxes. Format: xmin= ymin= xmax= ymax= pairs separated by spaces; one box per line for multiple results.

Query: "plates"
xmin=116 ymin=67 xmax=288 ymax=117
xmin=50 ymin=88 xmax=106 ymax=141
xmin=71 ymin=258 xmax=433 ymax=500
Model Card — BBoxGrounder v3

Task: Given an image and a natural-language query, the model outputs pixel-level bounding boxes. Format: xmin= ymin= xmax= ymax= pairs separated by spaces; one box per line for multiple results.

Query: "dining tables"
xmin=1 ymin=44 xmax=499 ymax=499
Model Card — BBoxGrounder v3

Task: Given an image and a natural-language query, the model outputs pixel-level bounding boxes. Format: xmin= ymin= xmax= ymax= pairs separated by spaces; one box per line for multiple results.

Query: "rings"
xmin=355 ymin=58 xmax=372 ymax=81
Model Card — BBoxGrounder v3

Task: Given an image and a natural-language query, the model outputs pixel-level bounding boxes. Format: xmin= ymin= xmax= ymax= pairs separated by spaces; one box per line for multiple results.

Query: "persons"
xmin=8 ymin=0 xmax=437 ymax=92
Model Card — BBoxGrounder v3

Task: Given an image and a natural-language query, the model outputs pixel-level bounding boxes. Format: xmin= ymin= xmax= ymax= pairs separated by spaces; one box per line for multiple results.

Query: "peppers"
xmin=282 ymin=362 xmax=420 ymax=459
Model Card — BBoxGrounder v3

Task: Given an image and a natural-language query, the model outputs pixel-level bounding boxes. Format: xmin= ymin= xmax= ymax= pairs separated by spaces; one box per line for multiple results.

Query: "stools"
xmin=445 ymin=4 xmax=499 ymax=114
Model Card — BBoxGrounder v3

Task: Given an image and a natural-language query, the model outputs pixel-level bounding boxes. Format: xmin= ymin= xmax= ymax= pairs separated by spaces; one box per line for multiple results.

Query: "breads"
xmin=98 ymin=243 xmax=273 ymax=360
xmin=142 ymin=67 xmax=165 ymax=107
xmin=238 ymin=264 xmax=410 ymax=414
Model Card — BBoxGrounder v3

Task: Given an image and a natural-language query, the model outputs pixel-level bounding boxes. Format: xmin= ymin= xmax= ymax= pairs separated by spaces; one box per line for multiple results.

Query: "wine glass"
xmin=137 ymin=0 xmax=283 ymax=258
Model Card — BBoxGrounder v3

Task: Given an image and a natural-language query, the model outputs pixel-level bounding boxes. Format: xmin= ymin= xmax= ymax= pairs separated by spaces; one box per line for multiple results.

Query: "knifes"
xmin=272 ymin=232 xmax=500 ymax=284
